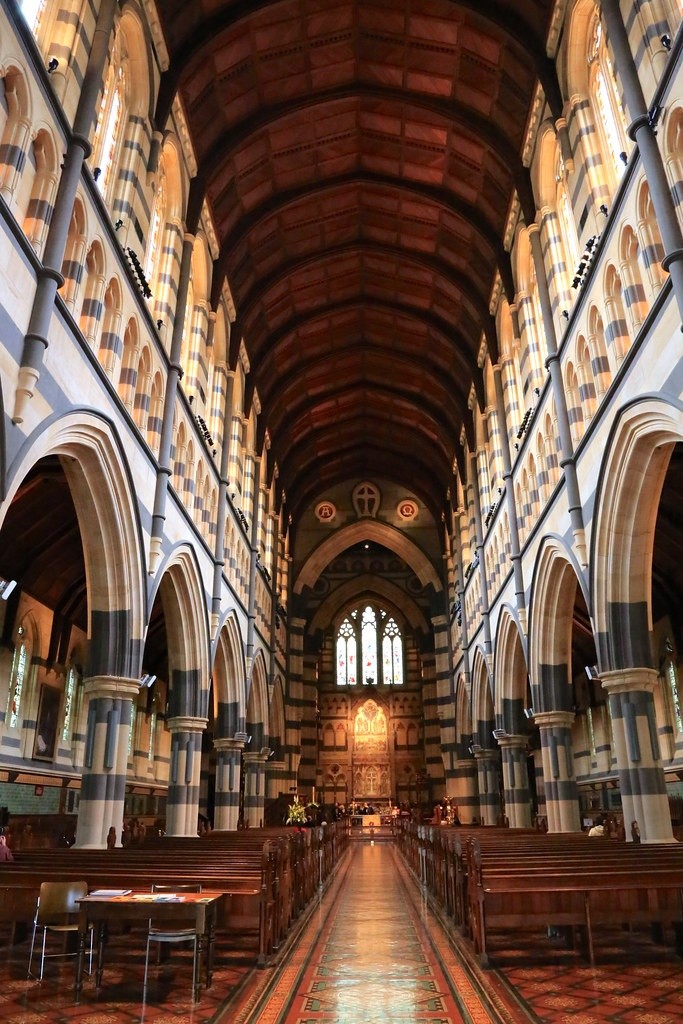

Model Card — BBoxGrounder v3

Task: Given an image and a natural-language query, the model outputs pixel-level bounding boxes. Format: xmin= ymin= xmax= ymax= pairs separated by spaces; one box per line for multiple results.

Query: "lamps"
xmin=141 ymin=673 xmax=156 ymax=688
xmin=233 ymin=731 xmax=252 ymax=743
xmin=524 ymin=708 xmax=533 ymax=719
xmin=0 ymin=579 xmax=17 ymax=600
xmin=492 ymin=729 xmax=506 ymax=739
xmin=584 ymin=665 xmax=600 ymax=680
xmin=261 ymin=747 xmax=275 ymax=756
xmin=468 ymin=745 xmax=481 ymax=753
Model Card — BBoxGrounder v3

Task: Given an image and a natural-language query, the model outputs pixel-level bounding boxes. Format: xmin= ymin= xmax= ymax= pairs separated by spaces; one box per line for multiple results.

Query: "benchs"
xmin=0 ymin=816 xmax=683 ymax=969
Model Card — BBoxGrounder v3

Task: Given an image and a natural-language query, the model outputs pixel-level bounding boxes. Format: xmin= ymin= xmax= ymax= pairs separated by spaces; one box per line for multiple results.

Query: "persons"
xmin=0 ymin=826 xmax=14 ymax=862
xmin=589 ymin=816 xmax=604 ymax=836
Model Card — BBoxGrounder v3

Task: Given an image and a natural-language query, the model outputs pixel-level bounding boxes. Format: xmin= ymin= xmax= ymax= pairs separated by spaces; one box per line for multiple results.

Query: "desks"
xmin=362 ymin=815 xmax=381 ymax=826
xmin=73 ymin=890 xmax=223 ymax=1003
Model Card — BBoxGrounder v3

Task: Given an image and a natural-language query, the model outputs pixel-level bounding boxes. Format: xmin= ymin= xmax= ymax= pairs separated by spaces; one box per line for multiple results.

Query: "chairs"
xmin=142 ymin=882 xmax=203 ymax=984
xmin=27 ymin=879 xmax=95 ymax=980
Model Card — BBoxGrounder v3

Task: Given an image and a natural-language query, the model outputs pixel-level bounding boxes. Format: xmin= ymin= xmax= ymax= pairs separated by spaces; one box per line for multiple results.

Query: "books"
xmin=90 ymin=890 xmax=132 ymax=896
